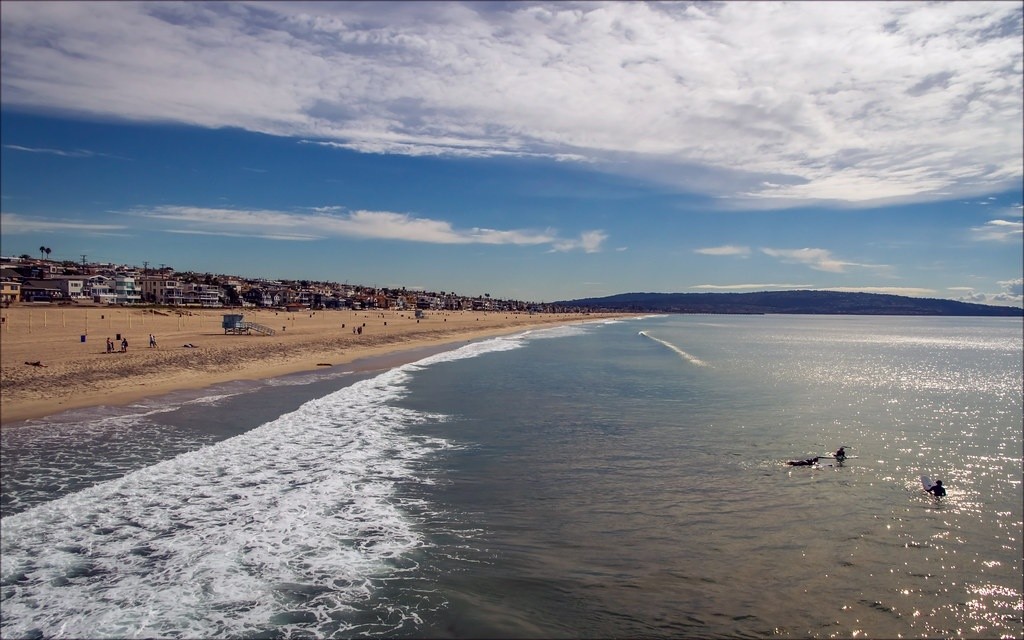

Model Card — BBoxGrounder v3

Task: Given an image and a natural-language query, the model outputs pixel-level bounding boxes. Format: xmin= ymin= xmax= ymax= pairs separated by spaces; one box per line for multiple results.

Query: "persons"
xmin=925 ymin=480 xmax=947 ymax=497
xmin=150 ymin=334 xmax=159 ymax=348
xmin=120 ymin=337 xmax=129 ymax=352
xmin=106 ymin=337 xmax=112 ymax=352
xmin=836 ymin=447 xmax=845 ymax=457
xmin=352 ymin=326 xmax=362 ymax=334
